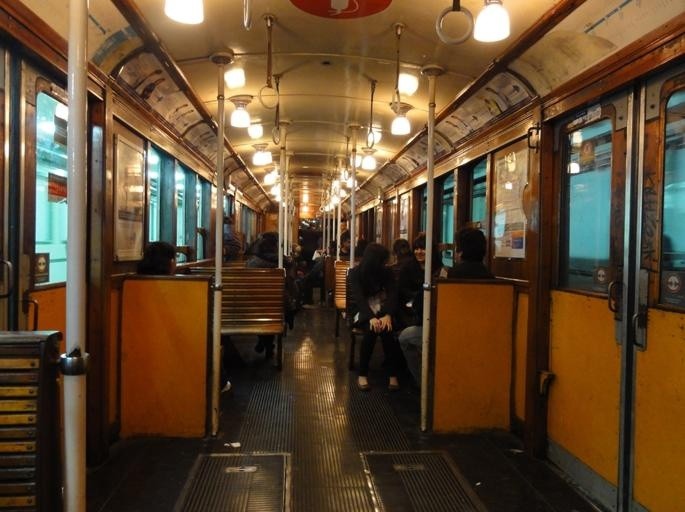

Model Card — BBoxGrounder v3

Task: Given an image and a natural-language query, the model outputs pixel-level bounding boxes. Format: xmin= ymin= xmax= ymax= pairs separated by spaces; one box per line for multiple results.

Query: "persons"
xmin=378 ymin=231 xmax=446 ymax=369
xmin=195 ymin=215 xmax=414 ymax=365
xmin=343 ymin=241 xmax=402 ymax=393
xmin=399 ymin=224 xmax=498 ymax=389
xmin=133 ymin=240 xmax=233 ymax=396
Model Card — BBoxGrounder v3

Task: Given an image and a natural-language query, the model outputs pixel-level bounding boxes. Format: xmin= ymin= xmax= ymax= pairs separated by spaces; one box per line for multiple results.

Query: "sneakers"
xmin=220 ymin=381 xmax=231 ymax=394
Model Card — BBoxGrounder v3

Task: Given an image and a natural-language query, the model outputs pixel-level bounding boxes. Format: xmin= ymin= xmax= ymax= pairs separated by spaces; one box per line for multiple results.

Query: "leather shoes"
xmin=356 ymin=375 xmax=371 ymax=391
xmin=388 ymin=377 xmax=400 ymax=392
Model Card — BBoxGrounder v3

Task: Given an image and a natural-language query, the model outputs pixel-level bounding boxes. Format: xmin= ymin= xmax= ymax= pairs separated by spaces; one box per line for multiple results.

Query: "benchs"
xmin=184 ymin=243 xmax=287 ymax=367
xmin=324 ymin=250 xmax=398 ymax=370
